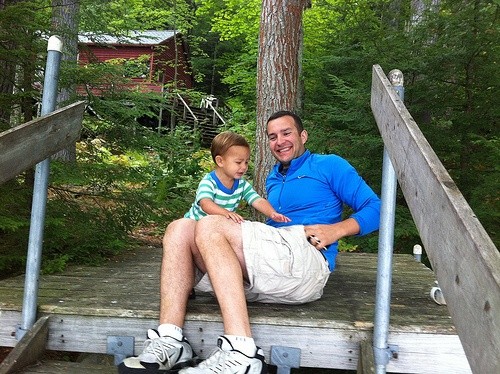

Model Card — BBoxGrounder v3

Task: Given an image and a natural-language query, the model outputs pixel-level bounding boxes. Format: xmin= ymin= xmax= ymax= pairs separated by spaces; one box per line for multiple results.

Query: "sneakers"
xmin=178 ymin=335 xmax=266 ymax=374
xmin=117 ymin=329 xmax=199 ymax=374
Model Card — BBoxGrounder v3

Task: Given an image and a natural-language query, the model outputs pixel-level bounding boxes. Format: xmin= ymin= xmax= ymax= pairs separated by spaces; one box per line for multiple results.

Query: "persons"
xmin=184 ymin=131 xmax=292 ymax=299
xmin=118 ymin=110 xmax=382 ymax=374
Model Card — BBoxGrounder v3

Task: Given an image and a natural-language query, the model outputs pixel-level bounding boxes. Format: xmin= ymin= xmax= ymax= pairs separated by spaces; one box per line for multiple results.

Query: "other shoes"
xmin=188 ymin=288 xmax=196 ymax=299
